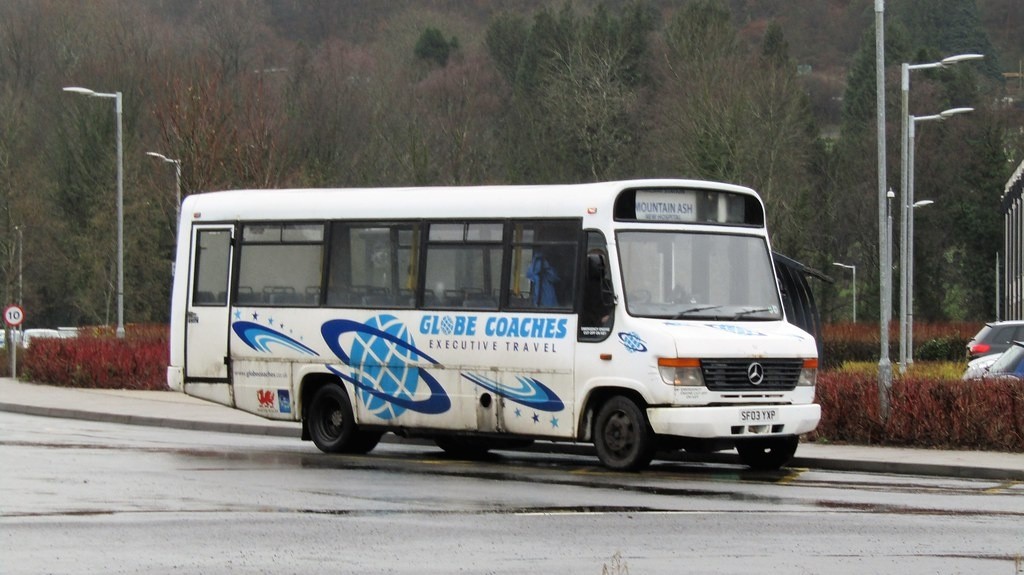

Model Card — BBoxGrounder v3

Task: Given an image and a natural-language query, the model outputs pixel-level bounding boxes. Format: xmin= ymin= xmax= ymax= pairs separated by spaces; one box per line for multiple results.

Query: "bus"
xmin=167 ymin=178 xmax=822 ymax=473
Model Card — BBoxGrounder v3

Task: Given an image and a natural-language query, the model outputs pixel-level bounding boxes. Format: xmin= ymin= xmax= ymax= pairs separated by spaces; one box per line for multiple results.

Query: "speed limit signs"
xmin=5 ymin=304 xmax=24 ymax=326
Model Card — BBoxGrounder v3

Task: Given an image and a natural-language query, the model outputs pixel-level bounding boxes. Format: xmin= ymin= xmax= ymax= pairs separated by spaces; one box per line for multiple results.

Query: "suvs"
xmin=981 ymin=339 xmax=1024 ymax=379
xmin=965 ymin=320 xmax=1024 ymax=361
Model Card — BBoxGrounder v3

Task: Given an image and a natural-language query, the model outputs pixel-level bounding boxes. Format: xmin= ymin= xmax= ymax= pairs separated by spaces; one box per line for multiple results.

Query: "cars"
xmin=962 ymin=353 xmax=1005 ymax=379
xmin=0 ymin=327 xmax=89 ymax=350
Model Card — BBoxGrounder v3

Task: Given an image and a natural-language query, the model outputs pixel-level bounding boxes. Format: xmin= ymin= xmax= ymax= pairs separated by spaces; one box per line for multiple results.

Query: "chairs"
xmin=194 ymin=283 xmax=530 ymax=311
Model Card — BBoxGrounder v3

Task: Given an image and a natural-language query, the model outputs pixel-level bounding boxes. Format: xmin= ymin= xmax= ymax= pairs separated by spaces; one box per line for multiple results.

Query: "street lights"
xmin=885 ymin=185 xmax=934 ymax=321
xmin=62 ymin=87 xmax=123 ymax=339
xmin=900 ymin=53 xmax=985 ymax=373
xmin=907 ymin=107 xmax=975 ymax=365
xmin=833 ymin=262 xmax=856 ymax=323
xmin=15 ymin=225 xmax=23 ymax=331
xmin=146 ymin=152 xmax=184 ymax=246
